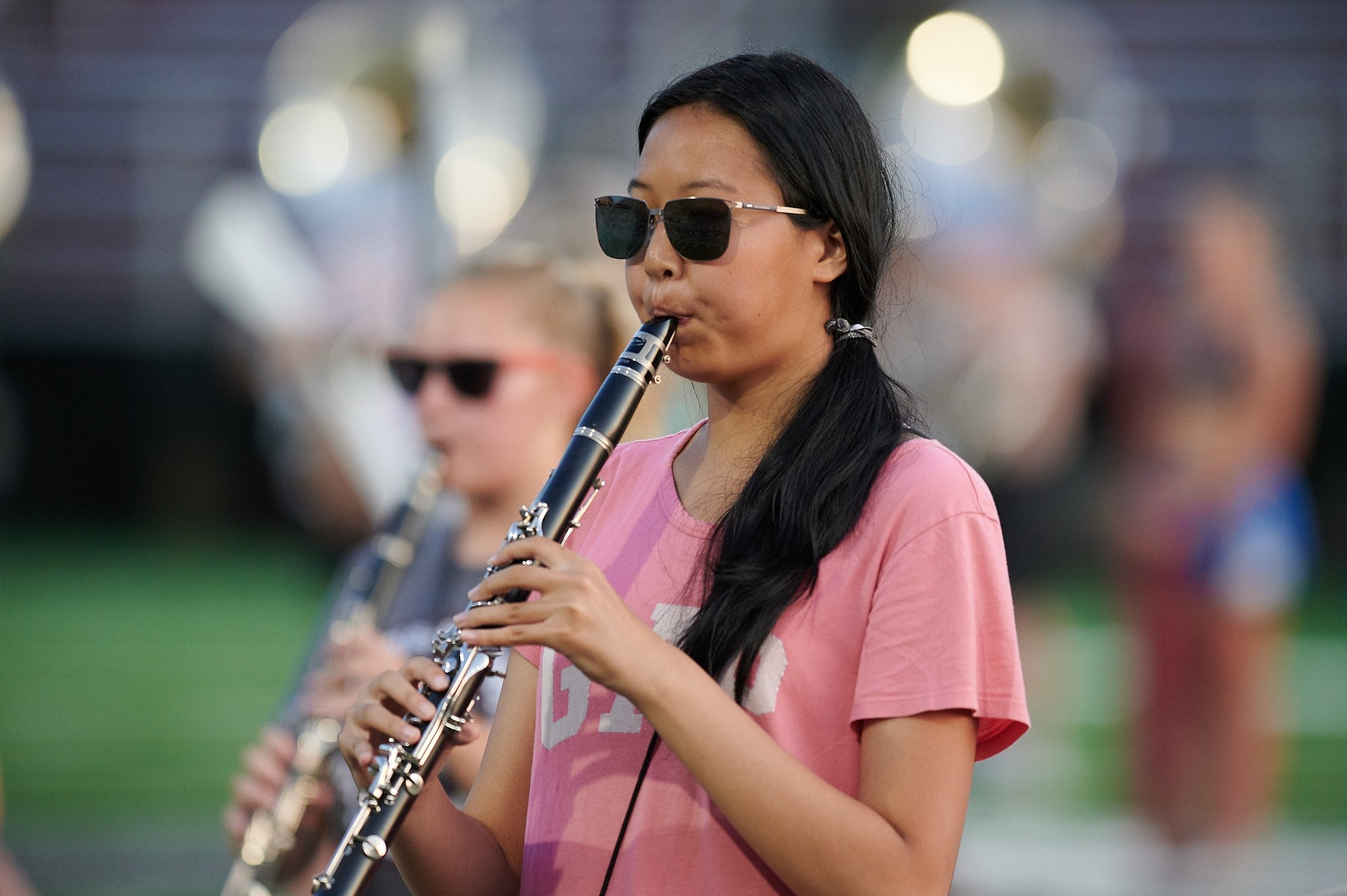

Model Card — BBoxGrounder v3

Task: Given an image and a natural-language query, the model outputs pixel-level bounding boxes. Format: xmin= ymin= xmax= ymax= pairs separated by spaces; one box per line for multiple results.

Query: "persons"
xmin=337 ymin=51 xmax=1032 ymax=895
xmin=1088 ymin=168 xmax=1319 ymax=851
xmin=223 ymin=241 xmax=659 ymax=895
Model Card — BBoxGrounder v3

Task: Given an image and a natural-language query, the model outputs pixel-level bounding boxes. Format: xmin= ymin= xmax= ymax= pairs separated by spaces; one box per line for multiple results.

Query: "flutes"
xmin=217 ymin=314 xmax=681 ymax=896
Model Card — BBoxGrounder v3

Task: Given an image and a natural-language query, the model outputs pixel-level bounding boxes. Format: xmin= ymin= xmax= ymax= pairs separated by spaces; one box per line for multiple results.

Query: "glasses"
xmin=390 ymin=356 xmax=501 ymax=398
xmin=594 ymin=195 xmax=808 ymax=264
